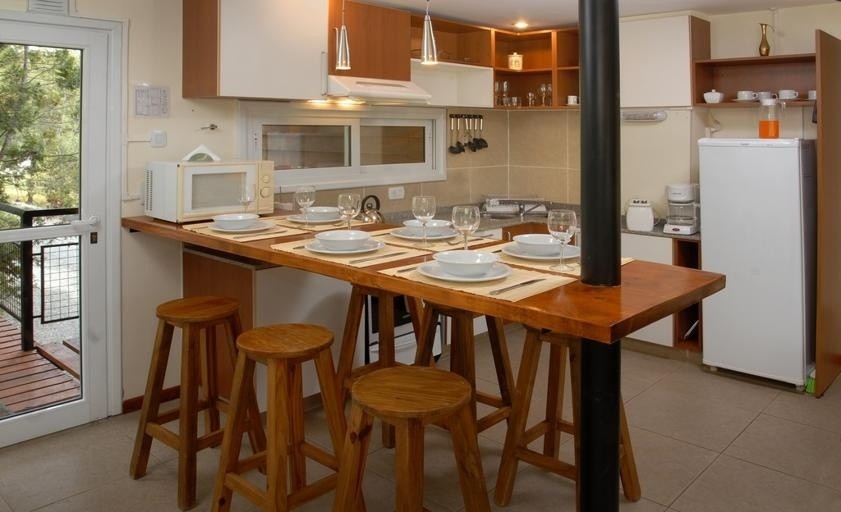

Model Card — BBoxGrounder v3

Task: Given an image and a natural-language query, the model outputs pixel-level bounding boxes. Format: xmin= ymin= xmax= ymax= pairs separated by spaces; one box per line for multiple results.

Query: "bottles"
xmin=508 ymin=52 xmax=524 ymax=71
xmin=759 ymin=22 xmax=771 ymax=57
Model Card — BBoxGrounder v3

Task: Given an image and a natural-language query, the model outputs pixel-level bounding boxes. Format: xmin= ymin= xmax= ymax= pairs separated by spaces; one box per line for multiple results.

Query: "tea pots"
xmin=759 ymin=99 xmax=787 ymax=139
xmin=351 ymin=196 xmax=386 ymax=224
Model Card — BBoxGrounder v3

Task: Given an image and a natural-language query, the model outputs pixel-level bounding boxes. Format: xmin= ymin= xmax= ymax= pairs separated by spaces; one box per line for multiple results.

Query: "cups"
xmin=703 ymin=87 xmax=817 ymax=103
xmin=568 ymin=95 xmax=578 ymax=104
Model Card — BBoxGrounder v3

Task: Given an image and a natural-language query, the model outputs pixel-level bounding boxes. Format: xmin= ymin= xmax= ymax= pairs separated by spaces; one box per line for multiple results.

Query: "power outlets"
xmin=440 ymin=227 xmax=503 ymax=344
xmin=705 ymin=125 xmax=712 ymax=138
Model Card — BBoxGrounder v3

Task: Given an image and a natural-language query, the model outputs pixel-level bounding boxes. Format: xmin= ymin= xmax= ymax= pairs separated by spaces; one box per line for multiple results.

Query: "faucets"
xmin=524 ymin=200 xmax=554 ymax=215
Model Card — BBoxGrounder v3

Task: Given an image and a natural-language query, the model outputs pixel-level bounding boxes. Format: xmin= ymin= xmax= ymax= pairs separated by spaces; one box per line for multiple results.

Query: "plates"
xmin=207 ymin=222 xmax=278 ymax=232
xmin=287 ymin=214 xmax=345 ymax=224
xmin=501 ymin=239 xmax=583 ymax=261
xmin=416 ymin=261 xmax=513 ymax=284
xmin=305 ymin=239 xmax=383 ymax=254
xmin=565 ymin=104 xmax=580 ymax=106
xmin=391 ymin=227 xmax=461 ymax=243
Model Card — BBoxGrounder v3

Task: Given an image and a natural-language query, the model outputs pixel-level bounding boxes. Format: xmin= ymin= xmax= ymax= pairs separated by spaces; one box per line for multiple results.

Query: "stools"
xmin=333 ymin=367 xmax=491 ymax=512
xmin=213 ymin=325 xmax=367 ymax=512
xmin=415 ymin=300 xmax=529 ymax=462
xmin=131 ymin=297 xmax=268 ymax=512
xmin=493 ymin=324 xmax=642 ymax=507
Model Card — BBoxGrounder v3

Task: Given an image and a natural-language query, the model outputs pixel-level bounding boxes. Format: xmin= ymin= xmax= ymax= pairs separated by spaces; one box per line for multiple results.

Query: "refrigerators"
xmin=696 ymin=136 xmax=816 ymax=393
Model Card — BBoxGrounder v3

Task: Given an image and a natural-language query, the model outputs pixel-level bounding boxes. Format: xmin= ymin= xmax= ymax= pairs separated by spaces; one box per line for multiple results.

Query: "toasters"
xmin=628 ymin=199 xmax=656 ymax=233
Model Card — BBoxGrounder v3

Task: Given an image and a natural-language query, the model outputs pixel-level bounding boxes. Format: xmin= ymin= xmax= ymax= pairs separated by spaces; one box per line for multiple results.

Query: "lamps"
xmin=420 ymin=3 xmax=439 ymax=65
xmin=513 ymin=19 xmax=526 ymax=27
xmin=335 ymin=3 xmax=351 ymax=70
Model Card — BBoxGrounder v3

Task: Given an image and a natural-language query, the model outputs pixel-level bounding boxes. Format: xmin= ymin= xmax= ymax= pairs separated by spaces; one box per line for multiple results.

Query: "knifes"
xmin=350 ymin=251 xmax=407 ymax=263
xmin=490 ymin=278 xmax=546 ymax=295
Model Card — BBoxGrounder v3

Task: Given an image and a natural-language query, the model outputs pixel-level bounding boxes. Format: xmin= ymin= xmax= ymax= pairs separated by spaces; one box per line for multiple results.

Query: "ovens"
xmin=365 ymin=290 xmax=443 ymax=368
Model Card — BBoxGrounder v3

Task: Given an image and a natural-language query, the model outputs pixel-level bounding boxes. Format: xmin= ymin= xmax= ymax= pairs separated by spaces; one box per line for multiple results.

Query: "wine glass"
xmin=295 ymin=185 xmax=317 ymax=230
xmin=495 ymin=80 xmax=553 ymax=107
xmin=451 ymin=205 xmax=480 ymax=251
xmin=339 ymin=194 xmax=362 ymax=228
xmin=547 ymin=209 xmax=577 ymax=274
xmin=414 ymin=196 xmax=439 ymax=249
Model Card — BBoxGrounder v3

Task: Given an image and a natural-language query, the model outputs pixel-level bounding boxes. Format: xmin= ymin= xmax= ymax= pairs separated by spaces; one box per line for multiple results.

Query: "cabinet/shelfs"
xmin=182 ymin=250 xmax=366 ymax=432
xmin=330 ymin=2 xmax=411 ymax=80
xmin=504 ymin=223 xmax=701 ymax=356
xmin=691 ymin=54 xmax=817 ymax=108
xmin=414 ymin=14 xmax=494 ymax=108
xmin=494 ymin=28 xmax=580 ymax=108
xmin=183 ymin=2 xmax=330 ymax=100
xmin=621 ymin=12 xmax=709 ymax=108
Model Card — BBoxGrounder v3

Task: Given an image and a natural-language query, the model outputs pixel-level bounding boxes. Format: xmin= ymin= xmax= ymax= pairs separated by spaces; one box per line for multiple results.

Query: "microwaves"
xmin=141 ymin=160 xmax=275 ymax=225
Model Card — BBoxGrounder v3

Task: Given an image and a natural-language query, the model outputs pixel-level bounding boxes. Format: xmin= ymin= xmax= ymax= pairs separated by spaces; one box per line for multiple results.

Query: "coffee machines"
xmin=663 ymin=184 xmax=701 ymax=238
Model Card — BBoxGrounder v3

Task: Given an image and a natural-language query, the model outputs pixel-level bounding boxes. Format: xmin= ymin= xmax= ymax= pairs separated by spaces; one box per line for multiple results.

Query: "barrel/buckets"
xmin=759 ymin=98 xmax=787 ymax=139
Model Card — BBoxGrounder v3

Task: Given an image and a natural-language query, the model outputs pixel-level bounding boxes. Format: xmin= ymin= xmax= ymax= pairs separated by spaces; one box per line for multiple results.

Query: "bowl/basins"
xmin=432 ymin=248 xmax=499 ymax=276
xmin=402 ymin=219 xmax=451 ymax=235
xmin=213 ymin=215 xmax=262 ymax=228
xmin=299 ymin=206 xmax=340 ymax=220
xmin=515 ymin=232 xmax=562 ymax=256
xmin=317 ymin=230 xmax=371 ymax=250
